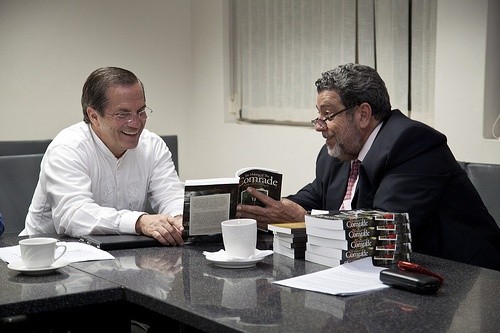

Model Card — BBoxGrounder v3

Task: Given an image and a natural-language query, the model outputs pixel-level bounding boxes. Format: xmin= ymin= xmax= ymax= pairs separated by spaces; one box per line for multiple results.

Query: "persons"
xmin=237 ymin=63 xmax=500 ymax=271
xmin=57 ymin=253 xmax=184 ymax=297
xmin=18 ymin=67 xmax=187 ymax=247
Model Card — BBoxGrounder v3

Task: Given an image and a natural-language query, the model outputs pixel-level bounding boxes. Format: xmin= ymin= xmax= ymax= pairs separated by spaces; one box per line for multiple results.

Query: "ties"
xmin=339 ymin=160 xmax=363 ymax=210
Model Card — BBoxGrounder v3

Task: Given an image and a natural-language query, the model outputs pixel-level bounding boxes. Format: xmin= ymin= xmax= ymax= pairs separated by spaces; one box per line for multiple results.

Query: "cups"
xmin=19 ymin=237 xmax=67 ymax=269
xmin=222 ymin=219 xmax=257 ymax=258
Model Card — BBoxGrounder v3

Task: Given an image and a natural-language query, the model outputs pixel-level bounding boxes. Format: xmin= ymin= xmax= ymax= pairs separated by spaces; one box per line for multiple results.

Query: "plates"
xmin=7 ymin=261 xmax=69 ymax=275
xmin=205 ymin=255 xmax=265 ymax=269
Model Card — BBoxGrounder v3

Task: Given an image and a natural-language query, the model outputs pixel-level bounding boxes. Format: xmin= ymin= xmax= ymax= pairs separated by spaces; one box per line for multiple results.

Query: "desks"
xmin=63 ymin=237 xmax=500 ymax=333
xmin=0 ymin=259 xmax=121 ymax=333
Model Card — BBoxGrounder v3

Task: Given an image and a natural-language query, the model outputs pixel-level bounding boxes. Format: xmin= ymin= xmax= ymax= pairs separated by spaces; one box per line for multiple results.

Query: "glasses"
xmin=96 ymin=106 xmax=153 ymax=123
xmin=312 ymin=103 xmax=363 ymax=129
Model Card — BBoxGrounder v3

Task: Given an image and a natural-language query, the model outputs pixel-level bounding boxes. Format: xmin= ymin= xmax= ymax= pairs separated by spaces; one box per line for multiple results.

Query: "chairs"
xmin=458 ymin=160 xmax=500 ymax=230
xmin=0 ymin=134 xmax=180 ymax=233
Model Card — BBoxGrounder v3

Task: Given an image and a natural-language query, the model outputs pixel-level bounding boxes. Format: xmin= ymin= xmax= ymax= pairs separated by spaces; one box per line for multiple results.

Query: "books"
xmin=305 ymin=291 xmax=376 ymax=319
xmin=183 ymin=167 xmax=412 ymax=267
